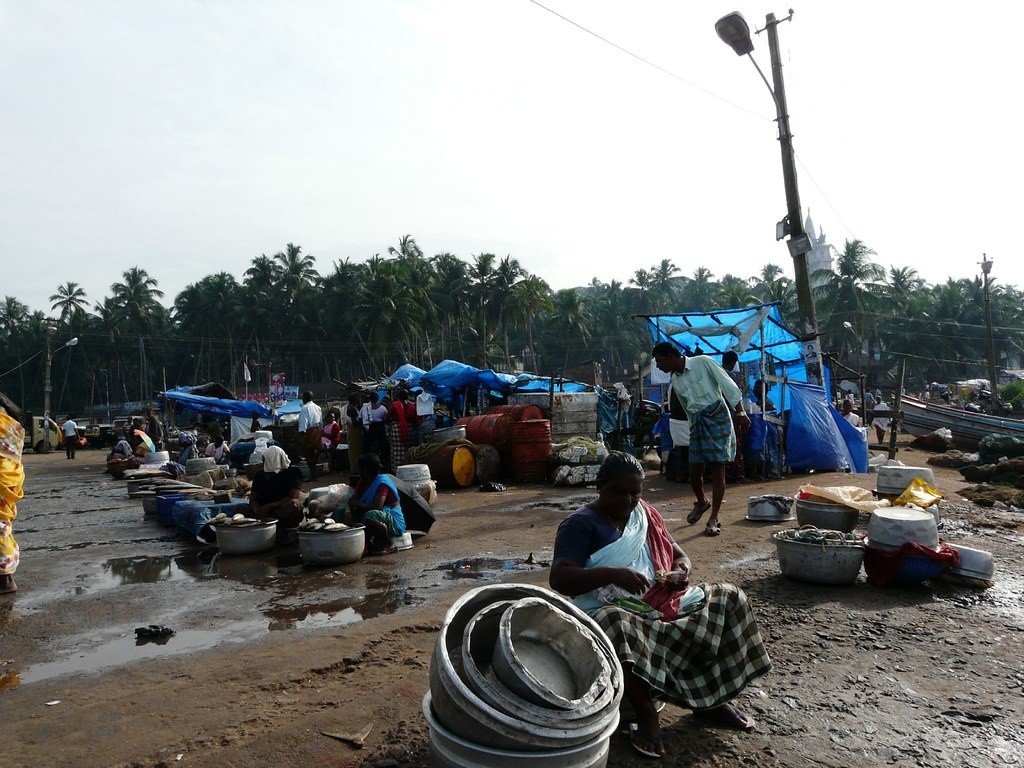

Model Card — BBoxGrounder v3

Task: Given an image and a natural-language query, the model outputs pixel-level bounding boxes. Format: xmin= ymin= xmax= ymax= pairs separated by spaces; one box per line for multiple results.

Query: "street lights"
xmin=982 ymin=260 xmax=1000 ymax=416
xmin=716 ymin=11 xmax=824 ymax=387
xmin=43 ymin=337 xmax=79 ymax=453
xmin=842 ymin=321 xmax=862 ymax=395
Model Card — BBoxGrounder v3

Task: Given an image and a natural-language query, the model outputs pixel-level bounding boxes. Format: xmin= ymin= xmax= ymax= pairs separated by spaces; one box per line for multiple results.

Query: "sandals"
xmin=687 ymin=497 xmax=712 ymax=523
xmin=706 ymin=518 xmax=721 ymax=534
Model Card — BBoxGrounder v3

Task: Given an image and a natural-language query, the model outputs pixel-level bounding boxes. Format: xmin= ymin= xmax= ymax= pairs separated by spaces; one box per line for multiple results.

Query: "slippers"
xmin=630 ymin=722 xmax=663 ymax=757
xmin=303 ymin=479 xmax=318 ymax=482
xmin=372 ymin=546 xmax=397 ymax=554
xmin=693 ymin=707 xmax=755 ymax=727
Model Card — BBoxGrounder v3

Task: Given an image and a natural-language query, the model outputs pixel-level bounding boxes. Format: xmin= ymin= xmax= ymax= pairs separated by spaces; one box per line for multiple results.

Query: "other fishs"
xmin=122 ymin=469 xmax=173 ymax=478
xmin=206 ymin=513 xmax=227 ymax=523
xmin=297 ymin=518 xmax=320 ymax=530
xmin=138 ymin=478 xmax=205 ymax=490
xmin=224 ymin=518 xmax=233 ymax=526
xmin=324 ymin=518 xmax=336 ymax=524
xmin=324 ymin=523 xmax=349 ymax=532
xmin=307 ymin=523 xmax=325 ymax=531
xmin=233 ymin=513 xmax=244 ymax=521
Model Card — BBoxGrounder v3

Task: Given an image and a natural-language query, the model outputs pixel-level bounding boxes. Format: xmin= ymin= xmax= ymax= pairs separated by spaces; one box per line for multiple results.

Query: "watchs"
xmin=736 ymin=411 xmax=747 ymax=417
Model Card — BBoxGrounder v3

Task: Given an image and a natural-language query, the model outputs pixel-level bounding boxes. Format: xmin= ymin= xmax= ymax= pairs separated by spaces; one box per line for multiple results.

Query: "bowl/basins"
xmin=792 ymin=492 xmax=859 ymax=530
xmin=943 ymin=541 xmax=995 ymax=582
xmin=225 ymin=467 xmax=238 ymax=478
xmin=143 ymin=451 xmax=170 ymax=465
xmin=211 ymin=519 xmax=282 ymax=552
xmin=435 ymin=424 xmax=469 ymax=439
xmin=310 ymin=488 xmax=336 ymax=502
xmin=745 ymin=494 xmax=796 ymax=521
xmin=863 ymin=502 xmax=941 ymax=589
xmin=186 ymin=458 xmax=218 ymax=476
xmin=295 ymin=523 xmax=366 ymax=564
xmin=421 ymin=583 xmax=625 ymax=768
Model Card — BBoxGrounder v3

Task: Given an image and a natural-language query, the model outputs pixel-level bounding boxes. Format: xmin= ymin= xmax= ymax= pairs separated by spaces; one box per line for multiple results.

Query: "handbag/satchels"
xmin=360 ymin=407 xmax=375 ymax=438
xmin=891 ymin=477 xmax=943 ymax=507
xmin=76 ymin=437 xmax=86 ymax=446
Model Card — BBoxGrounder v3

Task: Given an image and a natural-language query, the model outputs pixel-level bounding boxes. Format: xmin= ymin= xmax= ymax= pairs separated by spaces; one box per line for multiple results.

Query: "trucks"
xmin=22 ymin=416 xmax=62 ymax=454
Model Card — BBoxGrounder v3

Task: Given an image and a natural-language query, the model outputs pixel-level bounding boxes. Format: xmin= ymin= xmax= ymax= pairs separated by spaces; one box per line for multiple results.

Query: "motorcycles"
xmin=84 ymin=415 xmax=148 ymax=450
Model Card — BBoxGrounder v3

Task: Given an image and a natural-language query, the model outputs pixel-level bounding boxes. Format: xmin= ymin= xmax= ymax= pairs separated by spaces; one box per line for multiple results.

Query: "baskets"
xmin=901 ymin=556 xmax=944 ymax=578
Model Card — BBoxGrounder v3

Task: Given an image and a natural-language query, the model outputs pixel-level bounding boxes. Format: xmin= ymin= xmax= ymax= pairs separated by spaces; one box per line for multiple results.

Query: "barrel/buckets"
xmin=511 ymin=418 xmax=556 ymax=458
xmin=455 ymin=404 xmax=543 ymax=445
xmin=407 ymin=445 xmax=499 ymax=484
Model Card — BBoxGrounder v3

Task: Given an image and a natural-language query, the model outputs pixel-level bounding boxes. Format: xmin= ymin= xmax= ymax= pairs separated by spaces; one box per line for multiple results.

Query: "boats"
xmin=891 ymin=391 xmax=1024 ymax=452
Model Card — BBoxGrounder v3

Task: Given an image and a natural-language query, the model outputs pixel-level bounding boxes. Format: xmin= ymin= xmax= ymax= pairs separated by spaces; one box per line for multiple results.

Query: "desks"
xmin=652 ymin=409 xmax=780 ymax=480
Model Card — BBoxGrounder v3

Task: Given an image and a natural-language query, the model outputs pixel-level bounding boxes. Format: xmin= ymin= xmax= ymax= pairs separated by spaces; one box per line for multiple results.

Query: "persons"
xmin=298 ymin=390 xmax=322 ymax=482
xmin=250 ymin=446 xmax=304 ymax=529
xmin=321 ymin=412 xmax=339 ymax=460
xmin=204 ymin=435 xmax=230 ymax=465
xmin=875 ymin=387 xmax=931 ymax=405
xmin=865 ymin=388 xmax=876 ymax=409
xmin=653 ymin=342 xmax=752 ymax=534
xmin=107 ymin=408 xmax=163 ymax=460
xmin=62 ymin=416 xmax=81 ymax=459
xmin=750 ymin=380 xmax=777 ymax=411
xmin=720 ymin=349 xmax=739 ymax=383
xmin=551 ymin=454 xmax=773 ymax=756
xmin=845 ymin=389 xmax=854 ymax=406
xmin=344 ymin=451 xmax=405 ymax=554
xmin=872 ymin=395 xmax=892 ymax=444
xmin=838 ymin=399 xmax=861 ymax=427
xmin=339 ymin=389 xmax=436 ymax=478
xmin=835 ymin=389 xmax=841 ymax=399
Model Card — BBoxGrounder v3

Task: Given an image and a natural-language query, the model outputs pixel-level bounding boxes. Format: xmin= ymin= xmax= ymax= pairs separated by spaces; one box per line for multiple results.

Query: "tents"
xmin=360 ymin=299 xmax=870 ymax=473
xmin=159 ymin=382 xmax=306 ymax=446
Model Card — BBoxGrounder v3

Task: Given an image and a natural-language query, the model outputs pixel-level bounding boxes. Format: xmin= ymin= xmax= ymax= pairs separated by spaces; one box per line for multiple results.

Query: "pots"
xmin=772 ymin=525 xmax=864 ymax=585
xmin=872 ymin=466 xmax=938 ymax=502
